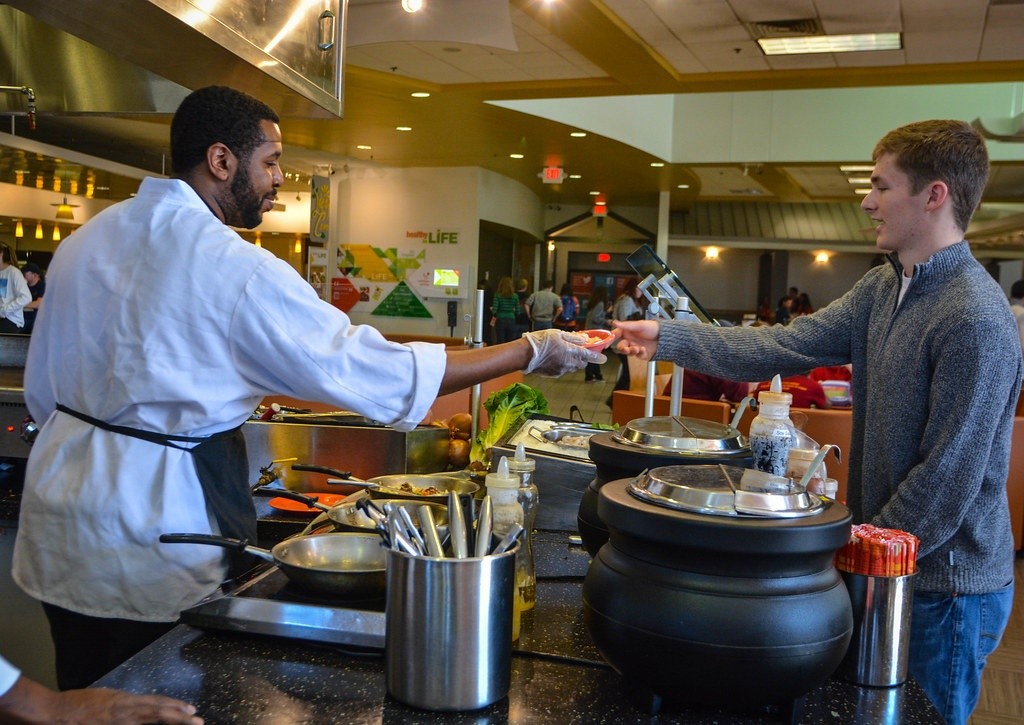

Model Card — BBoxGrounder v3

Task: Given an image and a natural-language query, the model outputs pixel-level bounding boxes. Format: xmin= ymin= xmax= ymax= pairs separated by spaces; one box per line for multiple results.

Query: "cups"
xmin=384 ymin=539 xmax=521 ymax=712
xmin=836 ymin=567 xmax=920 ymax=687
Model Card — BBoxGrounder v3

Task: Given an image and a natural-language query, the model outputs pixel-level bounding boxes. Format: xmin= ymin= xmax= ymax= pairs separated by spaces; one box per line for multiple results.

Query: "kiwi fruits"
xmin=447 ymin=413 xmax=471 ymax=467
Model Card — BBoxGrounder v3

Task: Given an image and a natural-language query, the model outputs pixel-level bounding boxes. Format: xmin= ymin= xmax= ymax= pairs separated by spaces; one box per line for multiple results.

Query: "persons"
xmin=11 ymin=86 xmax=610 ymax=693
xmin=612 ymin=119 xmax=1024 ymax=725
xmin=480 ymin=278 xmax=814 ymax=405
xmin=0 ymin=249 xmax=47 ymax=334
xmin=0 ymin=658 xmax=205 ymax=725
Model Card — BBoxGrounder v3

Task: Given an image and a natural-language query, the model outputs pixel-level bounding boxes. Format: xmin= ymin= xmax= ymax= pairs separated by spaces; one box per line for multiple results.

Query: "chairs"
xmin=612 ymin=389 xmax=853 ymax=505
xmin=1007 ymin=417 xmax=1024 ymax=550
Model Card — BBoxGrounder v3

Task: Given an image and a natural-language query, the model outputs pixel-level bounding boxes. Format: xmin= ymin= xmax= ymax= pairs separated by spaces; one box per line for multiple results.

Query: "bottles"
xmin=786 ymin=446 xmax=839 ymax=500
xmin=749 ymin=374 xmax=794 ymax=478
xmin=480 ymin=454 xmax=522 ymax=642
xmin=500 ymin=440 xmax=540 ymax=610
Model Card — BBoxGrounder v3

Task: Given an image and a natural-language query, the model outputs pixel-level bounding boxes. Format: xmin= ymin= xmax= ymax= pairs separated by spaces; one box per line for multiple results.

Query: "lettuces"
xmin=466 ymin=382 xmax=549 ymax=473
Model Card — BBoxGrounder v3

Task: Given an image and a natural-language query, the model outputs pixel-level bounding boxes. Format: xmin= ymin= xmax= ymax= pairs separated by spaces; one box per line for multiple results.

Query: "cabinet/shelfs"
xmin=0 ymin=0 xmax=350 ymax=123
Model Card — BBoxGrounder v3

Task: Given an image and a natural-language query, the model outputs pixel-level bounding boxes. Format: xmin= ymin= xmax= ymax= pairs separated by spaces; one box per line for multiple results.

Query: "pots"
xmin=291 ymin=461 xmax=481 ymax=510
xmin=253 ymin=483 xmax=453 ymax=536
xmin=158 ymin=530 xmax=411 ymax=602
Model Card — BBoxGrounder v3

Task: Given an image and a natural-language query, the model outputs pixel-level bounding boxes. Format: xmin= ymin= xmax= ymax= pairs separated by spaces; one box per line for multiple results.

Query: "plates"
xmin=267 ymin=493 xmax=352 ymax=516
xmin=574 ymin=328 xmax=615 ymax=352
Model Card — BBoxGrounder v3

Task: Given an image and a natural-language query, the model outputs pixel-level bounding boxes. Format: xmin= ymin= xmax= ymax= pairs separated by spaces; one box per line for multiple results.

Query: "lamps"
xmin=537 ymin=167 xmax=568 ymax=183
xmin=590 ymin=206 xmax=609 ymax=217
xmin=50 ymin=166 xmax=81 ymax=219
xmin=16 ymin=220 xmax=60 ymax=241
